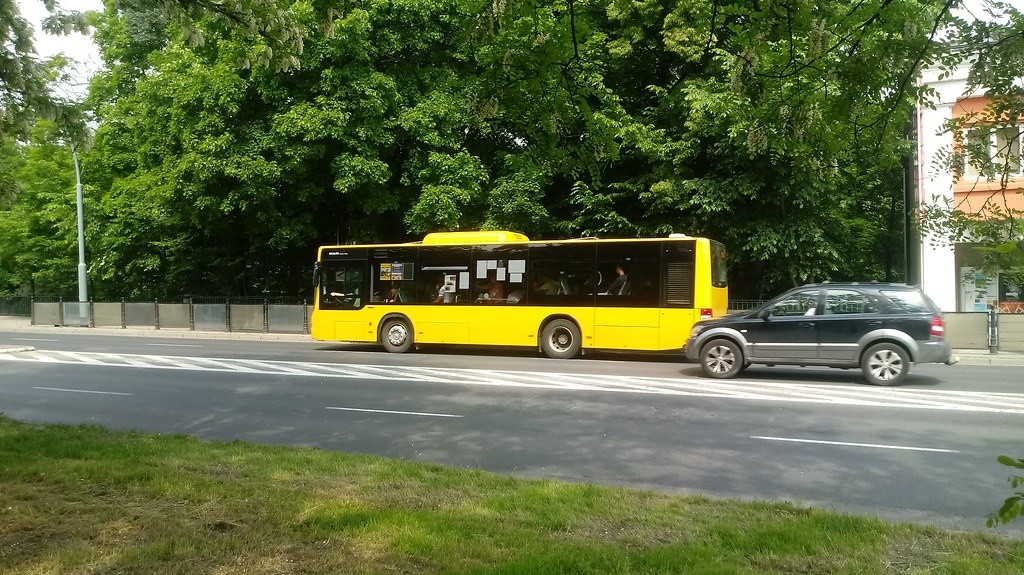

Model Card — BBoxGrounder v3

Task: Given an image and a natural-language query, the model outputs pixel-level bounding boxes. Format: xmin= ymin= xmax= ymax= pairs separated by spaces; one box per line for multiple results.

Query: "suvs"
xmin=681 ymin=279 xmax=961 ymax=388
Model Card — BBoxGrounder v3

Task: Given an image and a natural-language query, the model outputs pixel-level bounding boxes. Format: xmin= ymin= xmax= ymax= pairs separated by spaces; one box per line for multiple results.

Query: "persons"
xmin=589 ymin=263 xmax=631 ymax=296
xmin=387 ymin=271 xmax=560 ymax=305
xmin=803 ymin=299 xmax=816 ymax=316
xmin=346 ymin=284 xmax=361 ymax=308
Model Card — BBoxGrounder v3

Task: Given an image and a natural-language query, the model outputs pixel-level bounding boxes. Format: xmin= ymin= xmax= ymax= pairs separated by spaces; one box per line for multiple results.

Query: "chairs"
xmin=559 ymin=277 xmax=573 ymax=295
xmin=618 ymin=272 xmax=633 ymax=295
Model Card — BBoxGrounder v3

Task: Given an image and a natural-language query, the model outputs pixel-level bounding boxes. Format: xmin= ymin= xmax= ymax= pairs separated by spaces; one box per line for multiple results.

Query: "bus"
xmin=309 ymin=229 xmax=729 ymax=359
